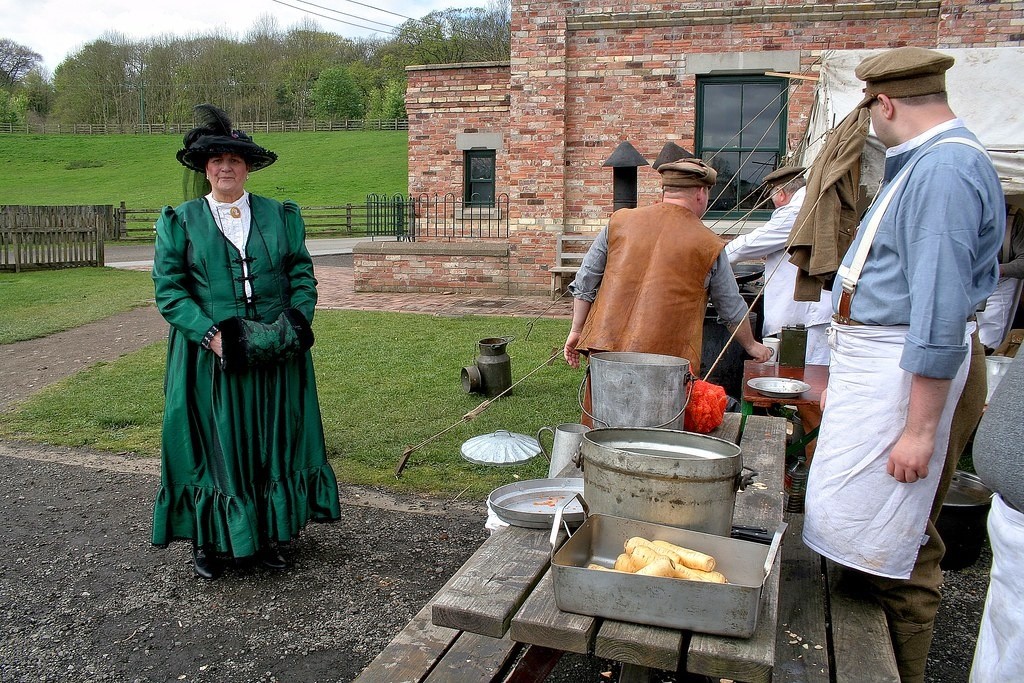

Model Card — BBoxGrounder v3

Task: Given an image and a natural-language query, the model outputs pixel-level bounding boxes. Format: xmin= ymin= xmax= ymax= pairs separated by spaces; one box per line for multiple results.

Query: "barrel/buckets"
xmin=789 ymin=410 xmax=805 ymax=445
xmin=571 ymin=426 xmax=759 ymax=538
xmin=578 ymin=352 xmax=698 ymax=431
xmin=477 ymin=335 xmax=516 ymax=397
xmin=934 ymin=470 xmax=995 ymax=570
xmin=985 ymin=356 xmax=1015 ymax=405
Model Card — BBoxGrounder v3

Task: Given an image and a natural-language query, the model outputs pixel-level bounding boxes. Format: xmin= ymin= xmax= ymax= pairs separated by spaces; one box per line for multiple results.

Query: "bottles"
xmin=784 ymin=456 xmax=810 ymax=513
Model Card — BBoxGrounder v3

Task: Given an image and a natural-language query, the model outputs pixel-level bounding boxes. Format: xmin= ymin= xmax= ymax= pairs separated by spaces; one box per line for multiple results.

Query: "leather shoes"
xmin=192 ymin=543 xmax=218 ymax=578
xmin=259 ymin=537 xmax=287 ymax=568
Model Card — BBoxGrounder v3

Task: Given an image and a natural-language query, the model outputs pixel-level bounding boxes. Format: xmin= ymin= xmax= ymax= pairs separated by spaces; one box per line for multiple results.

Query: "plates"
xmin=747 ymin=377 xmax=812 ymax=398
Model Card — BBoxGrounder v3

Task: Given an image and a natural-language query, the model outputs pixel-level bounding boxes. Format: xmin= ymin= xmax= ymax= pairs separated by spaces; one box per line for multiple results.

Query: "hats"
xmin=764 ymin=166 xmax=807 ymax=199
xmin=176 ymin=103 xmax=278 ymax=175
xmin=855 ymin=47 xmax=955 ymax=109
xmin=657 ymin=158 xmax=717 ymax=192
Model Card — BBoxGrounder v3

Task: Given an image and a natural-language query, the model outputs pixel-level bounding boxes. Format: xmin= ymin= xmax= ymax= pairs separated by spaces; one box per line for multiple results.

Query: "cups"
xmin=762 ymin=337 xmax=782 ymax=367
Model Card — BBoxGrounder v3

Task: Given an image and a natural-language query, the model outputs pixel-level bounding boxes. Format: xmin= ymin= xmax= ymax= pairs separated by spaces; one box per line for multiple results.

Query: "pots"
xmin=732 ymin=264 xmax=766 ymax=284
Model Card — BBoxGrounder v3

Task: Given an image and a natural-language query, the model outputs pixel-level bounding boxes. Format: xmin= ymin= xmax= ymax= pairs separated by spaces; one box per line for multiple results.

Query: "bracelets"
xmin=199 ymin=327 xmax=219 ymax=350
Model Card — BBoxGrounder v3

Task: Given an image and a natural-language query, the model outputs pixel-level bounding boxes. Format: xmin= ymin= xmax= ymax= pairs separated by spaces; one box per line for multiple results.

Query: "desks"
xmin=432 ymin=412 xmax=787 ymax=683
xmin=736 ymin=361 xmax=830 ymax=447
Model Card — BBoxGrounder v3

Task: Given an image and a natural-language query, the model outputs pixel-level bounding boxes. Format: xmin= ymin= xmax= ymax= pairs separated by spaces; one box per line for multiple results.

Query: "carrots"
xmin=588 ymin=537 xmax=730 ymax=585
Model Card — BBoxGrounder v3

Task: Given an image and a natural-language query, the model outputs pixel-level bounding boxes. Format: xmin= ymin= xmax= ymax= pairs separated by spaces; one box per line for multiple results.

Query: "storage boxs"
xmin=549 ymin=493 xmax=788 ymax=638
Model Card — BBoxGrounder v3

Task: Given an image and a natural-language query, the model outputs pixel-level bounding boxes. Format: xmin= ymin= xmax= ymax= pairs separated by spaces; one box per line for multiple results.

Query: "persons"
xmin=151 ymin=127 xmax=340 ymax=582
xmin=975 ymin=201 xmax=1023 ymax=357
xmin=799 ymin=43 xmax=1009 ymax=683
xmin=968 ymin=335 xmax=1023 ymax=683
xmin=719 ymin=167 xmax=839 ymax=469
xmin=563 ymin=156 xmax=772 ymax=431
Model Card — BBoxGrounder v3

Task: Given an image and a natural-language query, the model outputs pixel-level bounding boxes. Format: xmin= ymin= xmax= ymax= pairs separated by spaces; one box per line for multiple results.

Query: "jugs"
xmin=537 ymin=422 xmax=591 ymax=479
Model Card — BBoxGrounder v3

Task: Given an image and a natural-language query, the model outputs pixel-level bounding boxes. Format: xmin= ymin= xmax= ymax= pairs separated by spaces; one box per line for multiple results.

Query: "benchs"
xmin=772 ymin=512 xmax=901 ymax=683
xmin=548 ymin=232 xmax=596 ymax=300
xmin=352 ymin=526 xmax=563 ymax=683
